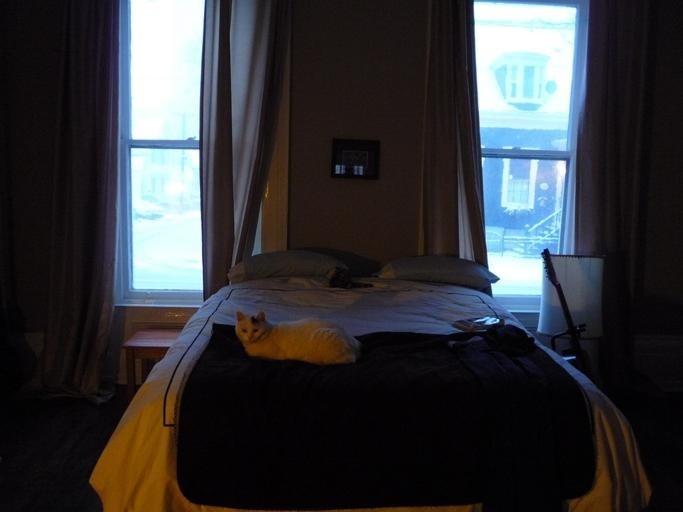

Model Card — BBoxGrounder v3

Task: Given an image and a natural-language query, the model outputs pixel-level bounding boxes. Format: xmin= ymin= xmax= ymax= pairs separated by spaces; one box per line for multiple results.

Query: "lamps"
xmin=534 ymin=251 xmax=606 ymax=380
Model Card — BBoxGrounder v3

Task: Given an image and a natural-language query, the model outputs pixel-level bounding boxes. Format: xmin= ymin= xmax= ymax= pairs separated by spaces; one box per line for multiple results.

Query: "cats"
xmin=235 ymin=310 xmax=359 ymax=365
xmin=325 ymin=266 xmax=373 ymax=289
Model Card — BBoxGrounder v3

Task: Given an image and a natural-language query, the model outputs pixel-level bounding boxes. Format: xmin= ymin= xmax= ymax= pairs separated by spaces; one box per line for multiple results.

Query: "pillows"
xmin=380 ymin=253 xmax=501 ymax=289
xmin=224 ymin=248 xmax=355 ymax=286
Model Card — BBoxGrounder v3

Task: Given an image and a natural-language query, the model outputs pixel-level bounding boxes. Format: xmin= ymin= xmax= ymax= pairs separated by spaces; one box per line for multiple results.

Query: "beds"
xmin=90 ymin=264 xmax=657 ymax=510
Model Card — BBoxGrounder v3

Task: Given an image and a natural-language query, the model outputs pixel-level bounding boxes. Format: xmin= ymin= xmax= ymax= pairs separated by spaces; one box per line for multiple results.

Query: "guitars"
xmin=542 ymin=249 xmax=603 ymax=391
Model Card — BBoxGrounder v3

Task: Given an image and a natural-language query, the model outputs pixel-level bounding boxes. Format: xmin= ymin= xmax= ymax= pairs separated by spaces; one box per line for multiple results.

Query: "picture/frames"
xmin=327 ymin=137 xmax=384 ymax=181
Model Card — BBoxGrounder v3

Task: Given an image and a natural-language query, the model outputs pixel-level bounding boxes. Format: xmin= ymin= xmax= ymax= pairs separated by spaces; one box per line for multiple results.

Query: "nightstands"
xmin=119 ymin=326 xmax=184 ymax=405
